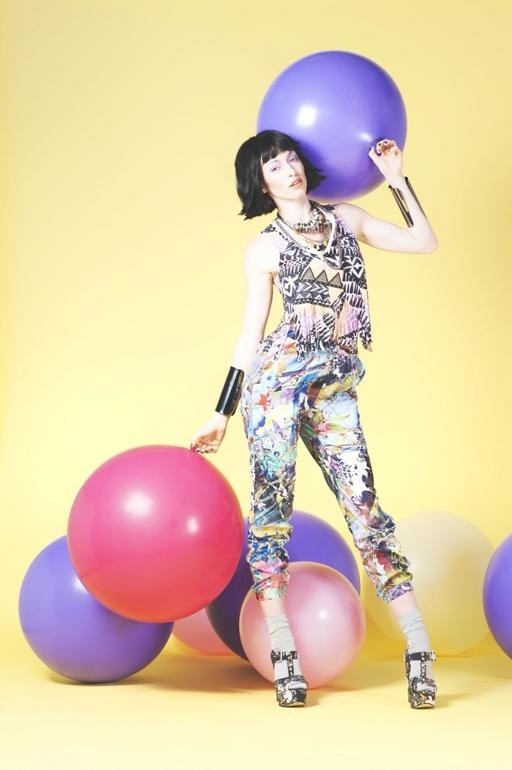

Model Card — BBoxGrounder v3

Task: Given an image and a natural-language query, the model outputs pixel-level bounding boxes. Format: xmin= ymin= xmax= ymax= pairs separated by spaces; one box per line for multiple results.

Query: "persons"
xmin=192 ymin=130 xmax=439 ymax=708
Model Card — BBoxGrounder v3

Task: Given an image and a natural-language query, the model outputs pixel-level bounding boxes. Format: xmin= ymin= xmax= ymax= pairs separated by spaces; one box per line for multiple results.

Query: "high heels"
xmin=404 ymin=649 xmax=437 ymax=709
xmin=271 ymin=650 xmax=308 ymax=707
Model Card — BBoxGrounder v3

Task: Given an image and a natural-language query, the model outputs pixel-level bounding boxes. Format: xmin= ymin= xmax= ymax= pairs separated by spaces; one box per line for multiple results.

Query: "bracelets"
xmin=389 ymin=176 xmax=427 ymax=228
xmin=215 ymin=366 xmax=244 ymax=416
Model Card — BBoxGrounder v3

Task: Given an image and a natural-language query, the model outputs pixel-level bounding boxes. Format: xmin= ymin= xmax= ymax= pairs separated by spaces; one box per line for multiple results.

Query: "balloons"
xmin=19 ymin=536 xmax=174 ymax=684
xmin=256 ymin=50 xmax=408 ymax=203
xmin=482 ymin=533 xmax=512 ymax=660
xmin=67 ymin=446 xmax=244 ymax=623
xmin=204 ymin=510 xmax=361 ymax=663
xmin=171 ymin=609 xmax=237 ymax=655
xmin=239 ymin=562 xmax=366 ymax=691
